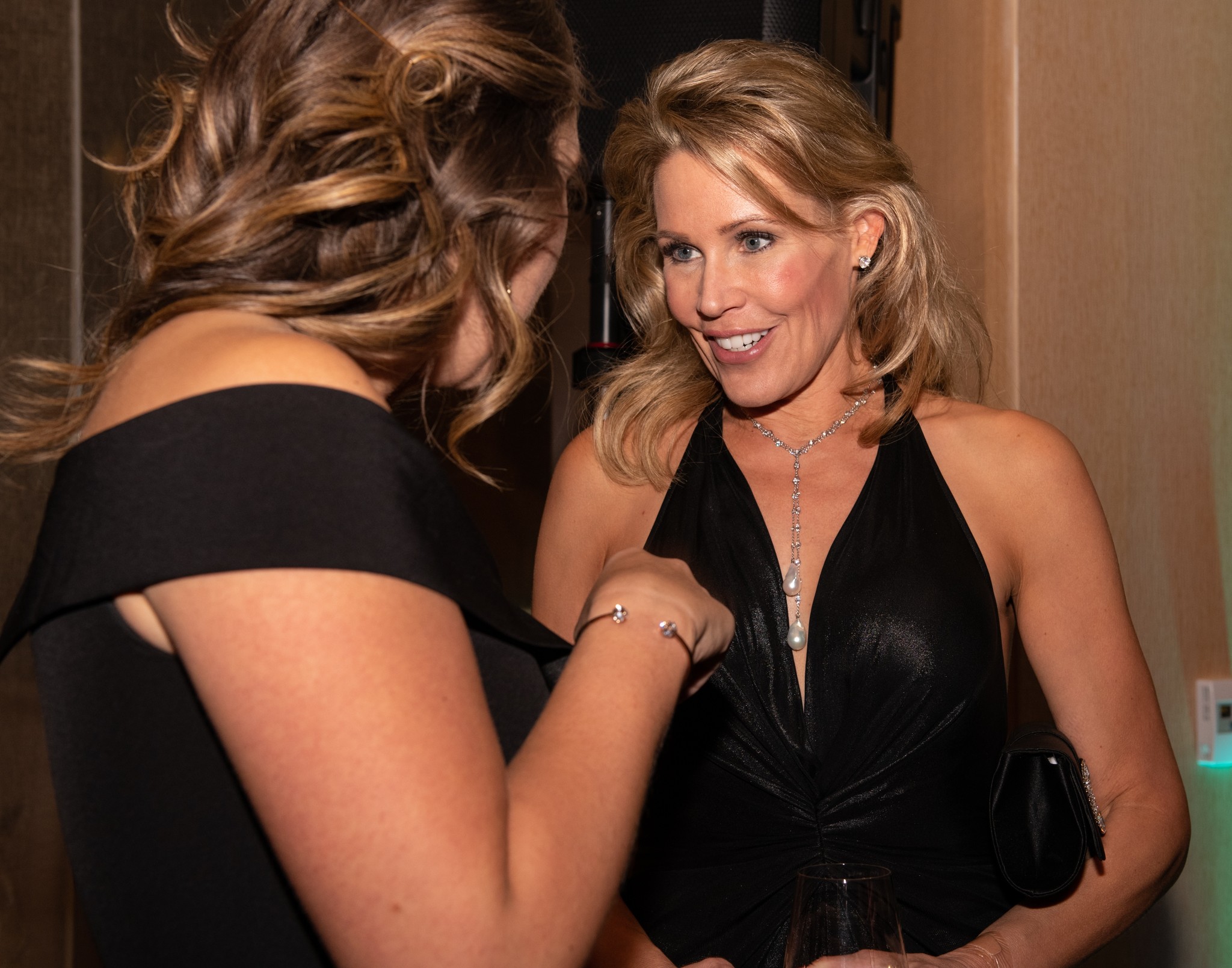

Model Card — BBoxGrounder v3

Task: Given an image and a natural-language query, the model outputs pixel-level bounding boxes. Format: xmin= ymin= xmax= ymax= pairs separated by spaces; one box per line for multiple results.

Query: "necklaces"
xmin=743 ymin=363 xmax=881 ymax=649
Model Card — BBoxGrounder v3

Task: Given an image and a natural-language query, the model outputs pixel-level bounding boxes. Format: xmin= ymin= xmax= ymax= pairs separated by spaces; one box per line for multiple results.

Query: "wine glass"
xmin=780 ymin=859 xmax=909 ymax=968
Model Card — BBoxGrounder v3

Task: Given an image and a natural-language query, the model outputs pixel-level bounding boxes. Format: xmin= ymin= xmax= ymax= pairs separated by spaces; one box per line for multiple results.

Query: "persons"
xmin=530 ymin=36 xmax=1194 ymax=968
xmin=0 ymin=0 xmax=741 ymax=968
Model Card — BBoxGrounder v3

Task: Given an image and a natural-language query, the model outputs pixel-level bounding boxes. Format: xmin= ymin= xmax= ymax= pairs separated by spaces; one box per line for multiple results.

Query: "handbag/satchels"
xmin=986 ymin=721 xmax=1105 ymax=910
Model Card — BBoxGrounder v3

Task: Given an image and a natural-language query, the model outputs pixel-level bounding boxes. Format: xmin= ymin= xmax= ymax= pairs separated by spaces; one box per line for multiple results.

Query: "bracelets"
xmin=574 ymin=604 xmax=694 ymax=679
